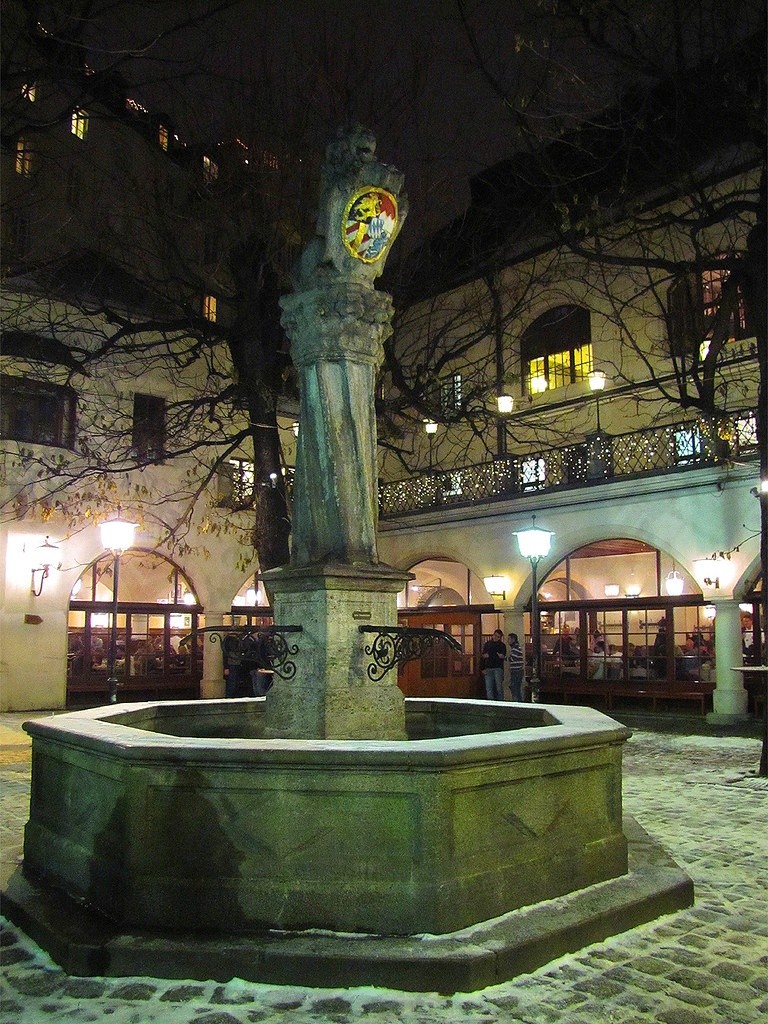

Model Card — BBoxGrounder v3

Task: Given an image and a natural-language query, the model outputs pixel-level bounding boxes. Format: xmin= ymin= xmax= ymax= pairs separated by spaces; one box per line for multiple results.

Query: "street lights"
xmin=512 ymin=515 xmax=556 ymax=704
xmin=587 ymin=368 xmax=606 ymax=429
xmin=422 ymin=417 xmax=438 ymax=469
xmin=497 ymin=393 xmax=514 ymax=452
xmin=97 ymin=505 xmax=141 ymax=705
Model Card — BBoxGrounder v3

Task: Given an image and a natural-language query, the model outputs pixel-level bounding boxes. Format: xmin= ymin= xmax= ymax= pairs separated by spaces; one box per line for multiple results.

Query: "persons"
xmin=496 ymin=633 xmax=525 ymax=702
xmin=480 ymin=629 xmax=506 ymax=701
xmin=68 ymin=625 xmax=275 ymax=679
xmin=539 ymin=612 xmax=767 ymax=690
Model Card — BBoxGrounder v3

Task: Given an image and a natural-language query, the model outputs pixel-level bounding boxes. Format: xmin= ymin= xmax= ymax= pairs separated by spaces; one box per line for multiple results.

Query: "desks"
xmin=730 ymin=664 xmax=768 ymax=718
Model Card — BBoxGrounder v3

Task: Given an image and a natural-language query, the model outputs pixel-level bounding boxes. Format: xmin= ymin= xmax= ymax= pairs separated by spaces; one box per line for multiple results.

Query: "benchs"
xmin=525 ymin=680 xmax=719 ymax=714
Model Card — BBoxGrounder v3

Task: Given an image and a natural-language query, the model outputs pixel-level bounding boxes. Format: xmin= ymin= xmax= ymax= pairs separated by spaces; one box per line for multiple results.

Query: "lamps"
xmin=484 ymin=575 xmax=510 ymax=602
xmin=37 ymin=536 xmax=58 ymax=568
xmin=693 ymin=559 xmax=736 ymax=590
